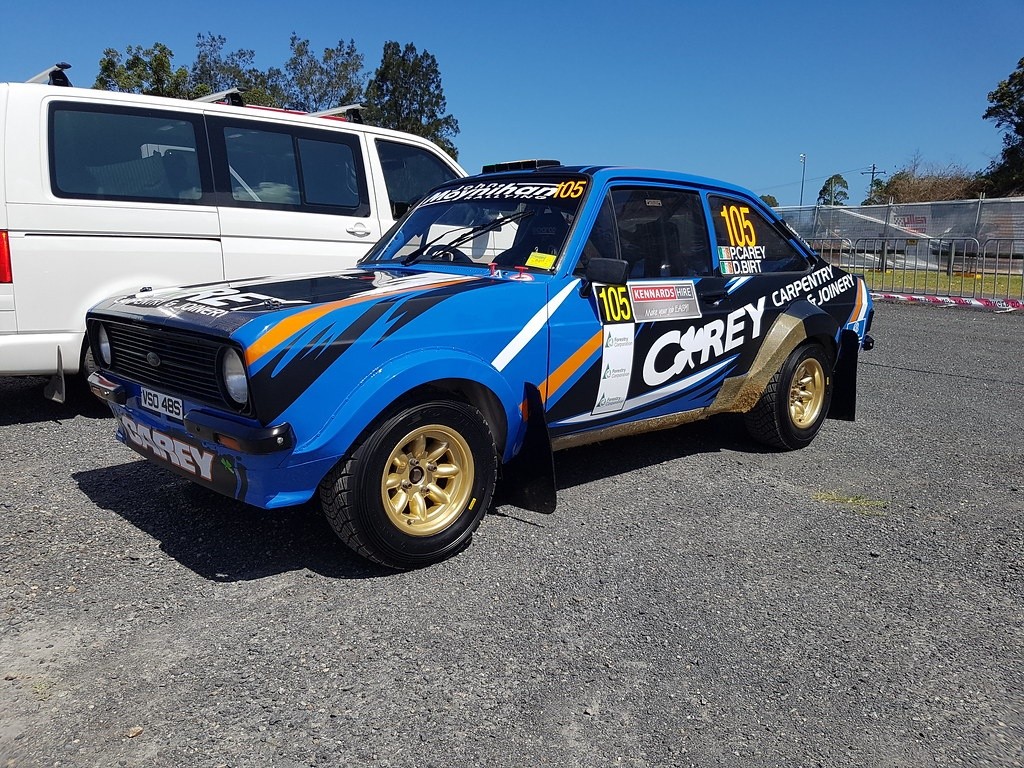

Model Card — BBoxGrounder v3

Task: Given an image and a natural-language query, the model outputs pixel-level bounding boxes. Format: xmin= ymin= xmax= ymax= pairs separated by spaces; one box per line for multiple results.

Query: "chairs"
xmin=621 ymin=219 xmax=693 ymax=280
xmin=492 ymin=212 xmax=569 ymax=270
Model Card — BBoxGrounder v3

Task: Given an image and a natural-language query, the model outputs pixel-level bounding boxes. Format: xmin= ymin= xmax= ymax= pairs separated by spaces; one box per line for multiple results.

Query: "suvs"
xmin=80 ymin=161 xmax=876 ymax=570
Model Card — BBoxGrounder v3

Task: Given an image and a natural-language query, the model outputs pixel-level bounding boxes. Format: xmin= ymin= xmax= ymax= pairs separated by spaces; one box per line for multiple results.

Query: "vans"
xmin=0 ymin=80 xmax=470 ymax=411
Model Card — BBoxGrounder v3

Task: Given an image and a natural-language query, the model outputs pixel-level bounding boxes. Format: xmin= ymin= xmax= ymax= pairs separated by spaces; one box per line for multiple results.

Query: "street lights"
xmin=798 ymin=153 xmax=805 ymax=231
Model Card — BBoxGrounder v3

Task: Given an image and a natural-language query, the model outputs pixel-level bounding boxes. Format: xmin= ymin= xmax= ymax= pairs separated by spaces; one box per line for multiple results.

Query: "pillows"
xmin=85 ymin=151 xmax=174 ymax=197
